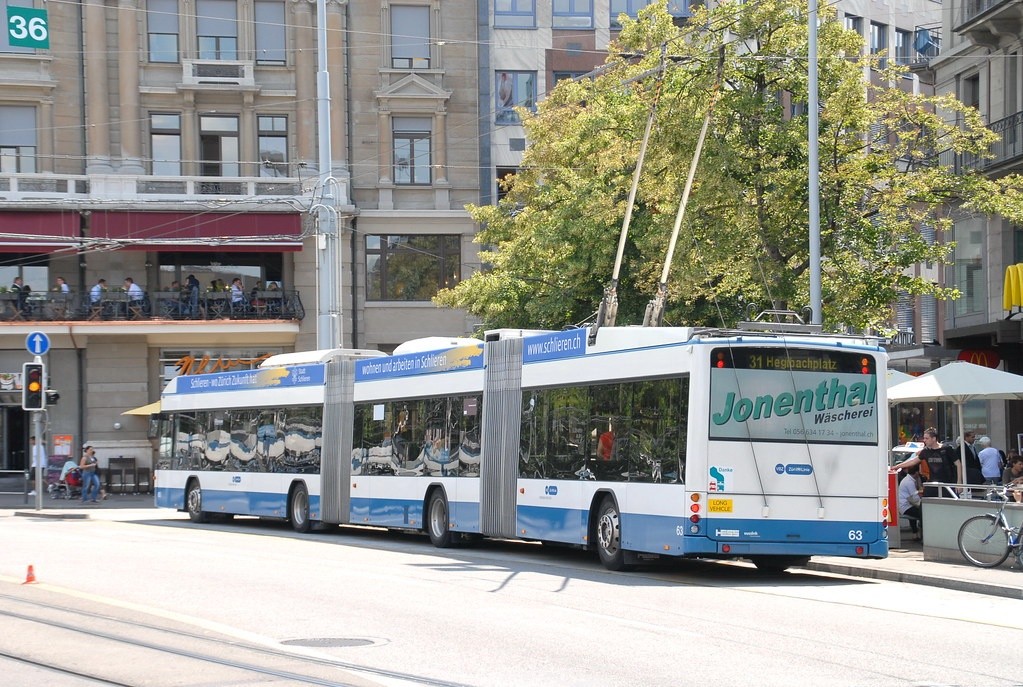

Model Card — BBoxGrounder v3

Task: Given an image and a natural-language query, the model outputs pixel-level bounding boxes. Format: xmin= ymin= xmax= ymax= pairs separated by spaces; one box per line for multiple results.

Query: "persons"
xmin=209 ymin=279 xmax=229 ymax=310
xmin=978 ymin=437 xmax=1023 ymax=503
xmin=90 ymin=279 xmax=110 ymax=321
xmin=10 ymin=277 xmax=22 ymax=313
xmin=17 ymin=285 xmax=31 ymax=322
xmin=80 ymin=446 xmax=100 ymax=504
xmin=250 ymin=281 xmax=265 ymax=308
xmin=51 ymin=277 xmax=70 ymax=302
xmin=229 ymin=278 xmax=250 ymax=320
xmin=897 ymin=466 xmax=923 ymax=545
xmin=919 ymin=459 xmax=929 ymax=483
xmin=58 ymin=453 xmax=82 ymax=490
xmin=121 ymin=278 xmax=144 ymax=321
xmin=165 ymin=274 xmax=200 ymax=320
xmin=270 ymin=282 xmax=282 ymax=291
xmin=890 ymin=427 xmax=962 ymax=499
xmin=27 ymin=436 xmax=54 ymax=496
xmin=955 ymin=430 xmax=987 ymax=500
xmin=596 ymin=413 xmax=628 ymax=459
xmin=92 ymin=451 xmax=106 ymax=500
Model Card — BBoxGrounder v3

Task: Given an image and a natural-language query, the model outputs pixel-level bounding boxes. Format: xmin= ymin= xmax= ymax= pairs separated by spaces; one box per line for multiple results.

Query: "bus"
xmin=151 ymin=308 xmax=889 ymax=572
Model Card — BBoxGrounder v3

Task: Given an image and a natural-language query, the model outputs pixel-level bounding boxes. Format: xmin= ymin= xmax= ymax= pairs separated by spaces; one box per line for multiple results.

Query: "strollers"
xmin=49 ymin=460 xmax=81 ymax=499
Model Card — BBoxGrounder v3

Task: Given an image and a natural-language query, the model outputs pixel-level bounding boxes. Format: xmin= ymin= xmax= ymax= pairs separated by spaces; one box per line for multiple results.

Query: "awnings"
xmin=0 ymin=210 xmax=303 ymax=252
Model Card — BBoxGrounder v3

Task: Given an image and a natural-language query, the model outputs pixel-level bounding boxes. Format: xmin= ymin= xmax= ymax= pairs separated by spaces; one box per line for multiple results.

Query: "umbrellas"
xmin=888 ymin=359 xmax=1023 ymax=499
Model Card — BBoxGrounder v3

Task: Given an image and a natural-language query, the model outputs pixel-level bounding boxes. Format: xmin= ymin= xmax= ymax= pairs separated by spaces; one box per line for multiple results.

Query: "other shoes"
xmin=27 ymin=490 xmax=35 ymax=495
xmin=83 ymin=500 xmax=90 ymax=504
xmin=912 ymin=537 xmax=921 ymax=541
xmin=90 ymin=498 xmax=100 ymax=502
xmin=101 ymin=495 xmax=107 ymax=500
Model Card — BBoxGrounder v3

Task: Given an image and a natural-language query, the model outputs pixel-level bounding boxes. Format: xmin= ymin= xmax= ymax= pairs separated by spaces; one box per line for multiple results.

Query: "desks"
xmin=256 ymin=291 xmax=284 ymax=317
xmin=150 ymin=292 xmax=183 ymax=317
xmin=199 ymin=292 xmax=232 ymax=319
xmin=100 ymin=292 xmax=132 ymax=319
xmin=21 ymin=295 xmax=48 ymax=320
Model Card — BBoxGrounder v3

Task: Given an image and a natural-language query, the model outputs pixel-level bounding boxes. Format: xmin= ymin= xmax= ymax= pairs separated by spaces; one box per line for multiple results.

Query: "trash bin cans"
xmin=887 ymin=471 xmax=902 ymax=549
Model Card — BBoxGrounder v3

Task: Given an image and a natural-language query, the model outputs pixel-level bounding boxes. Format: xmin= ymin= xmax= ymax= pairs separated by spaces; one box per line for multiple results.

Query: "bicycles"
xmin=957 ymin=481 xmax=1023 ymax=568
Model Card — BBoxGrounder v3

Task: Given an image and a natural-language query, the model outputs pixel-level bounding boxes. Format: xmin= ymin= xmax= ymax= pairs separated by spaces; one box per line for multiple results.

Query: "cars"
xmin=888 ymin=440 xmax=927 ymax=471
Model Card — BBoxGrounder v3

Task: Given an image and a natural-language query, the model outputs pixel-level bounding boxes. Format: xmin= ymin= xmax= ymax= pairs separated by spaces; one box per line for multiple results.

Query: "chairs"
xmin=0 ymin=289 xmax=286 ymax=321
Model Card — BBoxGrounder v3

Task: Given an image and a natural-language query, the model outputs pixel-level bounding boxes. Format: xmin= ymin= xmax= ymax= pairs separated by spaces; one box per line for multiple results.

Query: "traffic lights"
xmin=22 ymin=362 xmax=45 ymax=410
xmin=46 ymin=390 xmax=59 ymax=406
